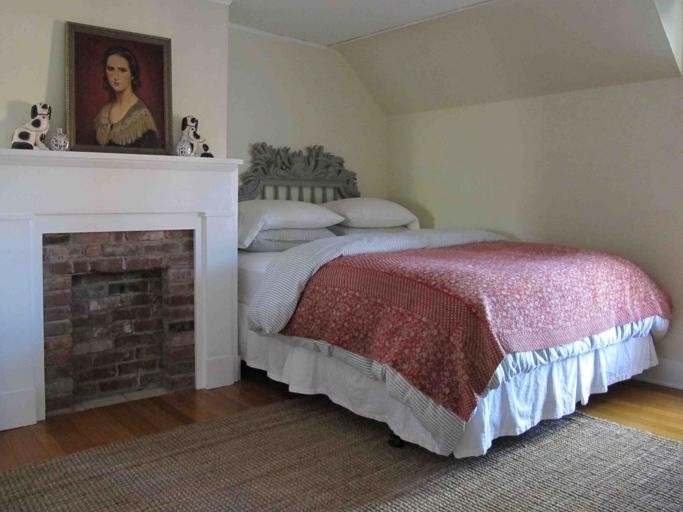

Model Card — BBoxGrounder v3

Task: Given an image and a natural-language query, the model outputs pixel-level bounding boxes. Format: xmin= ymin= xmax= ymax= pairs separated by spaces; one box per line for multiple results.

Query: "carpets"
xmin=2 ymin=397 xmax=683 ymax=512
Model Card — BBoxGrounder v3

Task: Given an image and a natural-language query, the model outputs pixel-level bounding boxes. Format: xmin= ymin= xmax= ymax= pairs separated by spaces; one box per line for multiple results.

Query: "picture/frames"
xmin=62 ymin=19 xmax=174 ymax=156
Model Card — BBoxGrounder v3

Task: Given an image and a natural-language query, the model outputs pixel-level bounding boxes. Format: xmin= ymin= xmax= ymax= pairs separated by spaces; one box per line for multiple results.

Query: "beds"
xmin=237 ymin=143 xmax=673 ymax=460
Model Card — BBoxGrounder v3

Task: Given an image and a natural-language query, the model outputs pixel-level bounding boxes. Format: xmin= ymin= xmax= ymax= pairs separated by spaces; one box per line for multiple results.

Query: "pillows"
xmin=327 ymin=224 xmax=411 ymax=235
xmin=320 ymin=196 xmax=421 ymax=230
xmin=238 ymin=199 xmax=345 ymax=249
xmin=238 ymin=228 xmax=337 ymax=253
